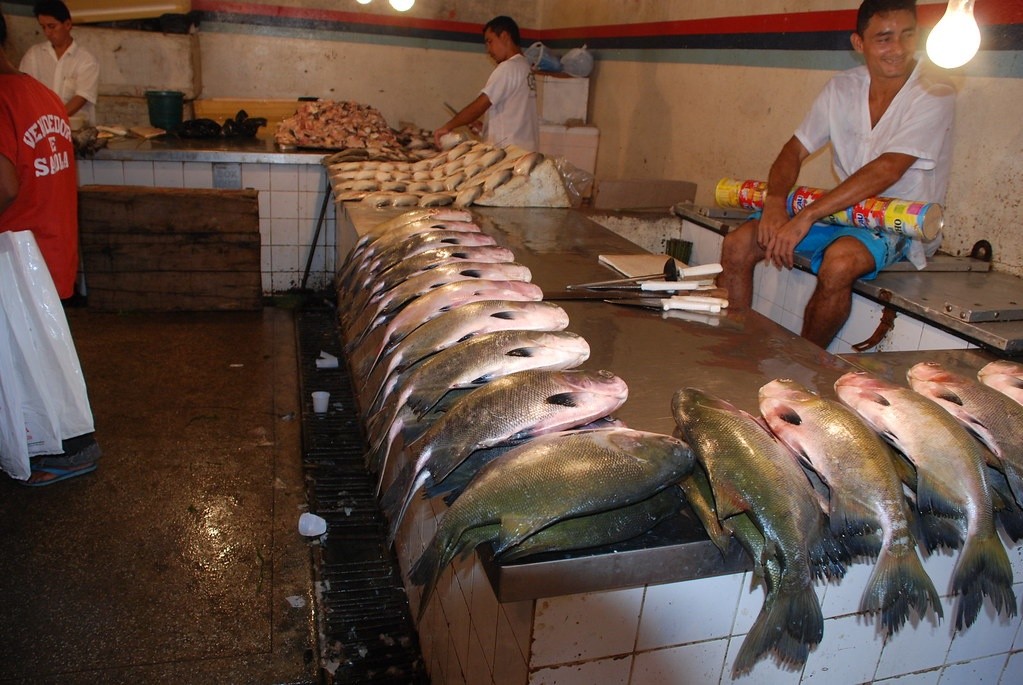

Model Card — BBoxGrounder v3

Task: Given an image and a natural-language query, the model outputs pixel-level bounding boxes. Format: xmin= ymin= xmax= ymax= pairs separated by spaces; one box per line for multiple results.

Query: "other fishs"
xmin=335 ymin=207 xmax=695 ymax=627
xmin=325 ymin=122 xmax=544 ymax=208
xmin=671 ymin=360 xmax=1023 ymax=681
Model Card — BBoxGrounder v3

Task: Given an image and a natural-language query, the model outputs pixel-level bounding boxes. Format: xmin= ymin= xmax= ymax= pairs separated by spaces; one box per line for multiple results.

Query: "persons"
xmin=716 ymin=1 xmax=955 ymax=351
xmin=434 ymin=15 xmax=539 ymax=153
xmin=0 ymin=13 xmax=101 ymax=486
xmin=20 ymin=0 xmax=97 ymax=128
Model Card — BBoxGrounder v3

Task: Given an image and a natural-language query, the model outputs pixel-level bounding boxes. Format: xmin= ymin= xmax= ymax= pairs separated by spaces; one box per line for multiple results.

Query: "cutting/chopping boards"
xmin=597 ymin=254 xmax=695 ymax=278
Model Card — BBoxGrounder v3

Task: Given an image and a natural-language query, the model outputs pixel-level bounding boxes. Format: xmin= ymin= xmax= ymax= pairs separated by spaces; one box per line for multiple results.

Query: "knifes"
xmin=542 ymin=262 xmax=730 ymax=312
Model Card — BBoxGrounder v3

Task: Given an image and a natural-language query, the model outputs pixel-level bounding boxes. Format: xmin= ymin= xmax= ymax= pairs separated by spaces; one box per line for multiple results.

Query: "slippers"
xmin=21 ymin=461 xmax=97 ymax=485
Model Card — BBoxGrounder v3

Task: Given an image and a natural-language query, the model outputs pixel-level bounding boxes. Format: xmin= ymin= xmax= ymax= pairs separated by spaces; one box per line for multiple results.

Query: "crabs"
xmin=275 ymin=98 xmax=399 ymax=153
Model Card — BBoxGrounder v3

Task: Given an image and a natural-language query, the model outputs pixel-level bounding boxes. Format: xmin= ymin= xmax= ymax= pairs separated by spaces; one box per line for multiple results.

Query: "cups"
xmin=298 ymin=513 xmax=327 ymax=536
xmin=312 ymin=391 xmax=331 ymax=413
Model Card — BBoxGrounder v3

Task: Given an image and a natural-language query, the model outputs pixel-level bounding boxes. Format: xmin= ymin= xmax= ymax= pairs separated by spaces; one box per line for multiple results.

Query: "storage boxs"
xmin=528 ymin=71 xmax=600 ymax=198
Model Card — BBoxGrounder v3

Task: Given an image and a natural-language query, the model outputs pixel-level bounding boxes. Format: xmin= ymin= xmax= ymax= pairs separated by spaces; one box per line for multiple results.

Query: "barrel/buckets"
xmin=144 ymin=91 xmax=185 ymax=132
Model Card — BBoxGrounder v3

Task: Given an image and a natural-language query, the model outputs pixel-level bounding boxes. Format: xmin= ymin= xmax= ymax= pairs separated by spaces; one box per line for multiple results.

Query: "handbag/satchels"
xmin=0 ymin=226 xmax=97 ymax=481
xmin=561 ymin=44 xmax=595 ymax=77
